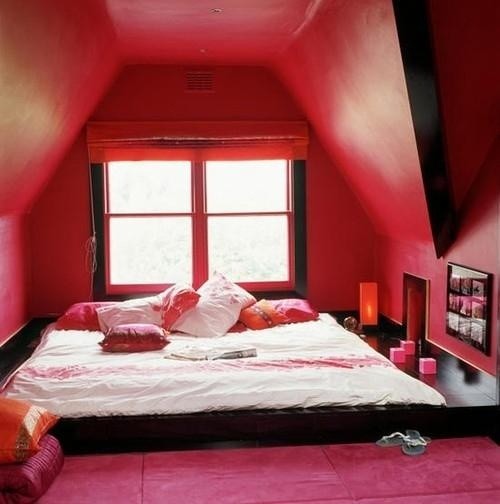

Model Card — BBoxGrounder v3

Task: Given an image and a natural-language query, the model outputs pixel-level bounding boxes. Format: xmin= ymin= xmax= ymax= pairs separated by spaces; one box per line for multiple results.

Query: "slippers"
xmin=403 ymin=430 xmax=427 ymax=455
xmin=376 ymin=432 xmax=432 ymax=447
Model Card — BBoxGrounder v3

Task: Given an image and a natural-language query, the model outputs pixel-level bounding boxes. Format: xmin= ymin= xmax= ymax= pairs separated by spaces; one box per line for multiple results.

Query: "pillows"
xmin=0 ymin=397 xmax=63 ymax=463
xmin=53 ymin=271 xmax=321 ymax=352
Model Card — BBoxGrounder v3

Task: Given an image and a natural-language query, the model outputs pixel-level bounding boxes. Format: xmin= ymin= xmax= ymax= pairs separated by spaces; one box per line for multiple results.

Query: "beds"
xmin=1 ymin=310 xmax=449 ymax=420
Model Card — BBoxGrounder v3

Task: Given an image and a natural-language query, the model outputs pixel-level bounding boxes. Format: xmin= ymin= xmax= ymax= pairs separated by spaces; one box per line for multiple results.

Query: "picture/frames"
xmin=446 ymin=262 xmax=493 ymax=354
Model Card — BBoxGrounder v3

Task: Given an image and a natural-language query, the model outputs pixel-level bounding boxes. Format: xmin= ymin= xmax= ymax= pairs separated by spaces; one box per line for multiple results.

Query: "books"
xmin=162 ymin=344 xmax=258 ymax=361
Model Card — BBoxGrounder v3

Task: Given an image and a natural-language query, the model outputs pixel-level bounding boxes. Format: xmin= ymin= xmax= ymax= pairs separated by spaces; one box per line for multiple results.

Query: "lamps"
xmin=358 ymin=280 xmax=378 ymax=333
